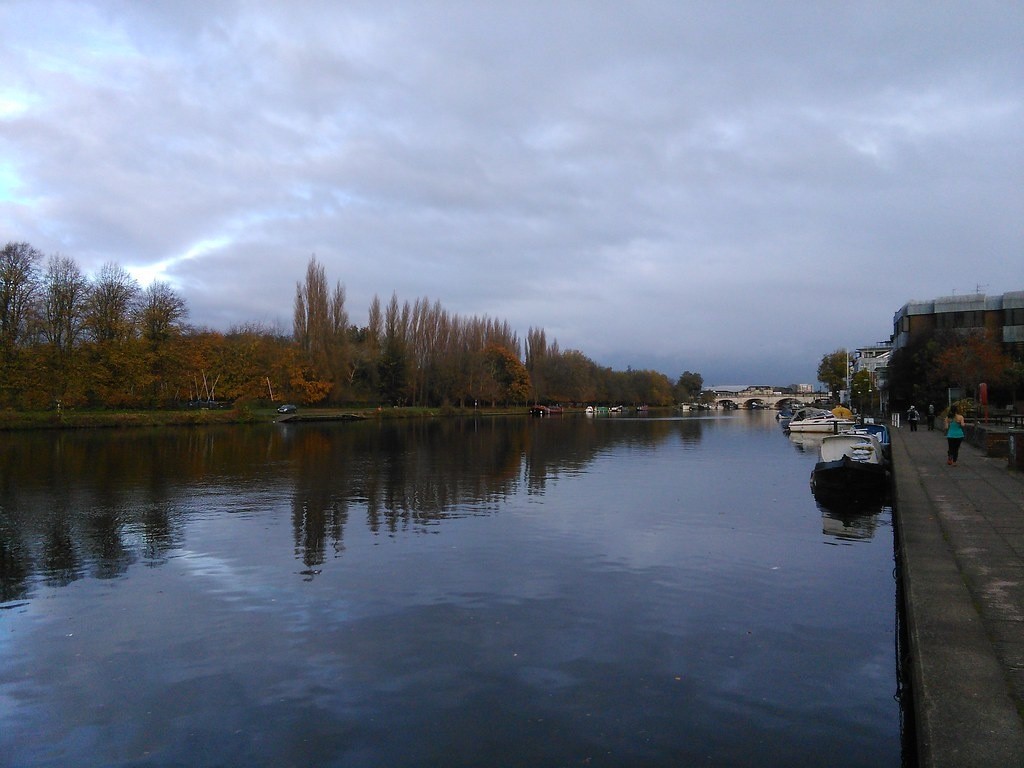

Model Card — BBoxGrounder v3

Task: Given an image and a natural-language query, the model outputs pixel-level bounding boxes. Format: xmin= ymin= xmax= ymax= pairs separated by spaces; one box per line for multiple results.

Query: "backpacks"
xmin=907 ymin=410 xmax=916 ymax=418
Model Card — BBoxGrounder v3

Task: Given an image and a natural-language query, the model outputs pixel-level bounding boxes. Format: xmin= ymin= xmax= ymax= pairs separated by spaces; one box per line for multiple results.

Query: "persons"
xmin=945 ymin=406 xmax=966 ymax=465
xmin=926 ymin=404 xmax=936 ymax=430
xmin=908 ymin=406 xmax=920 ymax=431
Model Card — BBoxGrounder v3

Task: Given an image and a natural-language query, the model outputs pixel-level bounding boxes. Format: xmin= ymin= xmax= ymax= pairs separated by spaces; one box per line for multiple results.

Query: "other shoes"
xmin=947 ymin=456 xmax=952 ymax=465
xmin=952 ymin=460 xmax=958 ymax=466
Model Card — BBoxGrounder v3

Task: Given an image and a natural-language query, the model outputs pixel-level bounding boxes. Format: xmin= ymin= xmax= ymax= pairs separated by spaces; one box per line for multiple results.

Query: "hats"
xmin=929 ymin=405 xmax=934 ymax=409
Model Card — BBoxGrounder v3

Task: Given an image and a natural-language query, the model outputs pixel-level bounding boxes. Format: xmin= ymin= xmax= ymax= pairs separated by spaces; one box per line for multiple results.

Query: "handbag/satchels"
xmin=943 ymin=416 xmax=951 ymax=429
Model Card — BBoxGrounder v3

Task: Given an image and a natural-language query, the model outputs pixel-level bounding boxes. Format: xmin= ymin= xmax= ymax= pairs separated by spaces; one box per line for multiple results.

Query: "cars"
xmin=278 ymin=405 xmax=297 ymax=414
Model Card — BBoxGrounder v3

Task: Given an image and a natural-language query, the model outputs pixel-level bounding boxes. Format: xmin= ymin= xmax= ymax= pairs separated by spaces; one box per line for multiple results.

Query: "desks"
xmin=1008 ymin=415 xmax=1024 ymax=429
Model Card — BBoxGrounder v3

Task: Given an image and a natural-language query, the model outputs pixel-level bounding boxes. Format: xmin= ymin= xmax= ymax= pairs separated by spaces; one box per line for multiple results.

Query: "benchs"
xmin=992 ymin=409 xmax=1008 ymax=426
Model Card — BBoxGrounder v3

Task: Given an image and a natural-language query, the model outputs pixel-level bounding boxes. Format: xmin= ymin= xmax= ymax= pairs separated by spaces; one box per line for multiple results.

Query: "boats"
xmin=847 ymin=424 xmax=889 ymax=459
xmin=638 ymin=406 xmax=657 ymax=411
xmin=815 ymin=497 xmax=887 ymax=544
xmin=809 ymin=434 xmax=891 ymax=495
xmin=789 ymin=407 xmax=855 ymax=433
xmin=584 ymin=405 xmax=629 ymax=412
xmin=778 ymin=407 xmax=794 ymax=420
xmin=529 ymin=405 xmax=563 ymax=415
